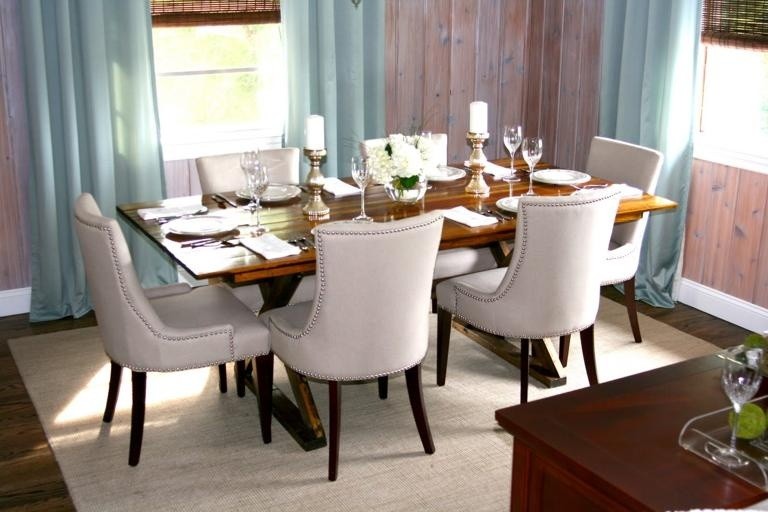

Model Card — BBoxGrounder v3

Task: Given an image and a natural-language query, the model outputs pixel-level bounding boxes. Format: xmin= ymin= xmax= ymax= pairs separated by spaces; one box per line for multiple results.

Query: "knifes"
xmin=215 ymin=192 xmax=237 ymax=207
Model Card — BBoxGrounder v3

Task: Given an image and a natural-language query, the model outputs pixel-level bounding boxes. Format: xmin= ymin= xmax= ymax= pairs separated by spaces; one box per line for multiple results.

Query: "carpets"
xmin=7 ymin=291 xmax=723 ymax=512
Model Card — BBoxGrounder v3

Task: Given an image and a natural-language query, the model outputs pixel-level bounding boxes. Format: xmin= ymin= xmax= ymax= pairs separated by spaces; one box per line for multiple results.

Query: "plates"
xmin=166 ymin=214 xmax=237 ymax=236
xmin=529 ymin=168 xmax=592 ymax=185
xmin=496 ymin=196 xmax=521 ymax=212
xmin=424 ymin=165 xmax=466 ymax=182
xmin=236 ymin=183 xmax=301 ymax=201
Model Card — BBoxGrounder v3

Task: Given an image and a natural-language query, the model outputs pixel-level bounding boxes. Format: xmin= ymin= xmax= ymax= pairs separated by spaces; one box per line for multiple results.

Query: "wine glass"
xmin=248 ymin=166 xmax=269 ymax=233
xmin=504 ymin=125 xmax=522 ymax=182
xmin=714 ymin=345 xmax=763 ymax=469
xmin=415 ymin=130 xmax=432 ymax=190
xmin=521 ymin=137 xmax=543 ymax=198
xmin=240 ymin=144 xmax=263 ymax=211
xmin=351 ymin=156 xmax=374 ymax=222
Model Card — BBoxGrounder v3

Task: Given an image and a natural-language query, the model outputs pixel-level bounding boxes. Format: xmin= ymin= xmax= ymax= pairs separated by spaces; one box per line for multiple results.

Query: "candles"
xmin=304 ymin=113 xmax=326 ymax=152
xmin=469 ymin=101 xmax=488 ymax=136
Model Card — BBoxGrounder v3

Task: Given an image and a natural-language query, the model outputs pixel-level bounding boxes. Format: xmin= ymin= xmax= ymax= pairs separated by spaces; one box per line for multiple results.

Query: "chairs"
xmin=70 ymin=196 xmax=275 ymax=466
xmin=360 ymin=132 xmax=498 ymax=316
xmin=251 ymin=210 xmax=446 ymax=483
xmin=434 ymin=186 xmax=621 ymax=404
xmin=558 ymin=135 xmax=665 ymax=368
xmin=192 ymin=146 xmax=317 ymax=391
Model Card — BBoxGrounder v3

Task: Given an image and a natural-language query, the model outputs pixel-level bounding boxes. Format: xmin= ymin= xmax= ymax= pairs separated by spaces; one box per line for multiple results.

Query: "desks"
xmin=494 ymin=345 xmax=768 ymax=511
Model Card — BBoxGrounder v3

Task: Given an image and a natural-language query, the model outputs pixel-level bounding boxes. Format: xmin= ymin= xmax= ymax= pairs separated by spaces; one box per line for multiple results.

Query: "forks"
xmin=570 ymin=184 xmax=608 ymax=190
